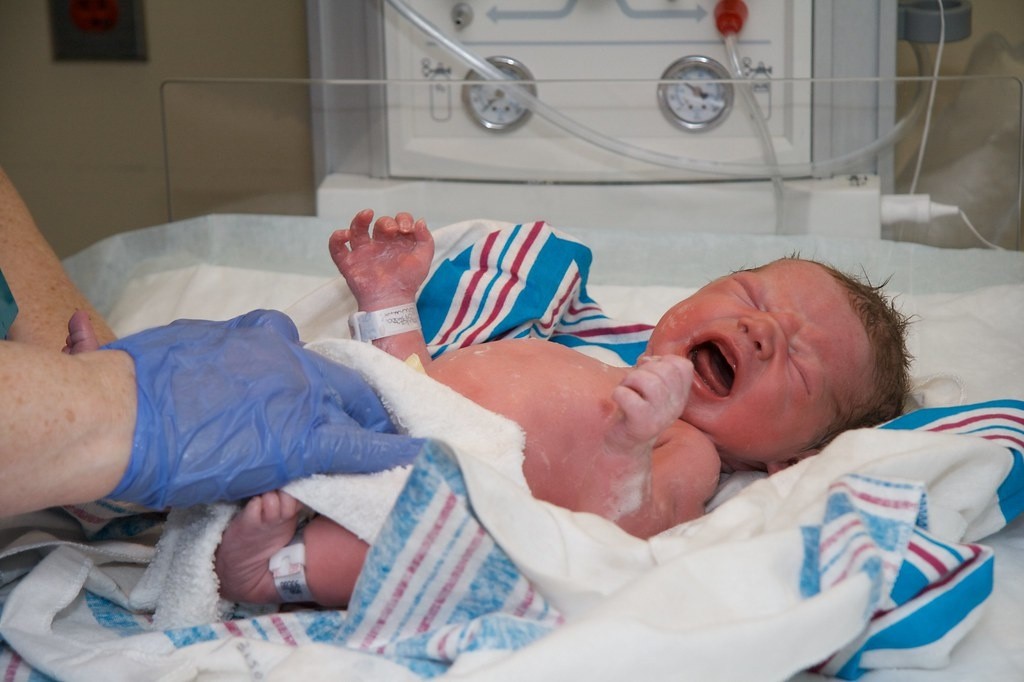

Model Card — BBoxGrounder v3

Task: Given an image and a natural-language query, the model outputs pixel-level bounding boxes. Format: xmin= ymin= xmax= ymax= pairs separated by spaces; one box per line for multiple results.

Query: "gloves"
xmin=101 ymin=306 xmax=427 ymax=510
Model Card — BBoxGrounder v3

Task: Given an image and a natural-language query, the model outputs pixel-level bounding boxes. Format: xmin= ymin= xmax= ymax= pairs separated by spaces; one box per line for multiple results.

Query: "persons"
xmin=62 ymin=208 xmax=912 ymax=606
xmin=0 ymin=165 xmax=417 ymax=513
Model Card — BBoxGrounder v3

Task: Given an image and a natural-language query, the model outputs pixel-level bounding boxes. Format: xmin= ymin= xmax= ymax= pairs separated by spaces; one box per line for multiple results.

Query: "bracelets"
xmin=346 ymin=300 xmax=419 ymax=343
